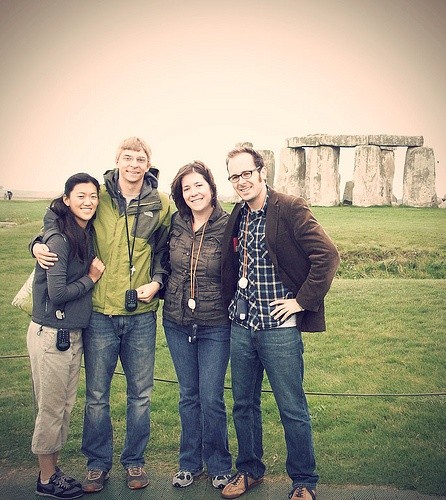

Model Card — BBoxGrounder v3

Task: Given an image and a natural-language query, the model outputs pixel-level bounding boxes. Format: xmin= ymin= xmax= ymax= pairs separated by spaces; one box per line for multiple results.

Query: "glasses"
xmin=228 ymin=167 xmax=260 ymax=183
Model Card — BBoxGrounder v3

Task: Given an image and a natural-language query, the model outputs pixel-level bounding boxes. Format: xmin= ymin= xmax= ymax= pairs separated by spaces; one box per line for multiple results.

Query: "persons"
xmin=27 ymin=137 xmax=176 ymax=492
xmin=27 ymin=173 xmax=106 ymax=500
xmin=8 ymin=191 xmax=12 ymax=200
xmin=161 ymin=161 xmax=233 ymax=488
xmin=220 ymin=142 xmax=340 ymax=500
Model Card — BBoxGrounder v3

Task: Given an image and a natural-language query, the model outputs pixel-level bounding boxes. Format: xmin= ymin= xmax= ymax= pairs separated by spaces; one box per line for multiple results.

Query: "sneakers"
xmin=127 ymin=466 xmax=148 ymax=489
xmin=172 ymin=467 xmax=205 ymax=488
xmin=213 ymin=474 xmax=231 ymax=488
xmin=53 ymin=465 xmax=81 ymax=488
xmin=81 ymin=468 xmax=109 ymax=492
xmin=36 ymin=472 xmax=83 ymax=500
xmin=221 ymin=472 xmax=264 ymax=498
xmin=288 ymin=486 xmax=316 ymax=500
